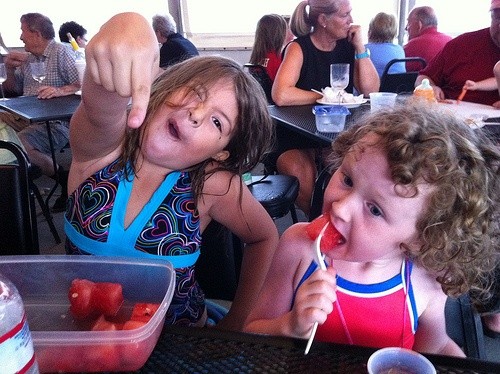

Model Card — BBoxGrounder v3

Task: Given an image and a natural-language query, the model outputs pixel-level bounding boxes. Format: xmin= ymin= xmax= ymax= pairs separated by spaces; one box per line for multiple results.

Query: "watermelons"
xmin=36 ymin=278 xmax=165 ymax=372
xmin=305 ymin=214 xmax=341 ymax=255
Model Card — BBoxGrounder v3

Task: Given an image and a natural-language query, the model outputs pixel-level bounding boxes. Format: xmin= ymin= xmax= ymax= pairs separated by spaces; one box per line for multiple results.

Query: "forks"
xmin=305 ymin=221 xmax=331 ymax=354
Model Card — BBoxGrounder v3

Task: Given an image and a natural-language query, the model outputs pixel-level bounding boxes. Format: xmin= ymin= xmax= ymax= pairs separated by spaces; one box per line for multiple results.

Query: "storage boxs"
xmin=312 ymin=104 xmax=353 ymax=133
xmin=0 ymin=256 xmax=177 ymax=374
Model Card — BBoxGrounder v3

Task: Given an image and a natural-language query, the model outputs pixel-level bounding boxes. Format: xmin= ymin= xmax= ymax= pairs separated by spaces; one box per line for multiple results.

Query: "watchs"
xmin=354 ymin=49 xmax=371 ymax=60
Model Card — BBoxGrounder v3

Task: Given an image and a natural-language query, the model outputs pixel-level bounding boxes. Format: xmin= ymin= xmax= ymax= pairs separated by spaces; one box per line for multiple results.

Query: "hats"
xmin=489 ymin=0 xmax=500 ymax=12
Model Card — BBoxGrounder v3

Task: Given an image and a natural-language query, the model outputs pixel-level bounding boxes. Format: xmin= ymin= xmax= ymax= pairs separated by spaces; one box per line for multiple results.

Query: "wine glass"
xmin=0 ymin=63 xmax=11 ymax=101
xmin=330 ymin=64 xmax=351 ymax=116
xmin=30 ymin=63 xmax=47 ymax=87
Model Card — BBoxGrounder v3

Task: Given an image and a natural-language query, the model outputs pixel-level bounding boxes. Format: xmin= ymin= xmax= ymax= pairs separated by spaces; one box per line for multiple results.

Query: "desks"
xmin=46 ymin=321 xmax=500 ymax=374
xmin=266 ymin=96 xmax=500 ymax=218
xmin=0 ymin=94 xmax=82 ymax=221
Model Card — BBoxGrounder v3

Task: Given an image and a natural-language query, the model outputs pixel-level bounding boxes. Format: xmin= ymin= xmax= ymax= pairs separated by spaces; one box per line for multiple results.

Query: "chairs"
xmin=245 ymin=174 xmax=301 ymax=225
xmin=307 ymin=163 xmax=488 ymax=362
xmin=378 ymin=56 xmax=428 ymax=94
xmin=0 ymin=140 xmax=41 ymax=256
xmin=242 ymin=63 xmax=274 ymax=104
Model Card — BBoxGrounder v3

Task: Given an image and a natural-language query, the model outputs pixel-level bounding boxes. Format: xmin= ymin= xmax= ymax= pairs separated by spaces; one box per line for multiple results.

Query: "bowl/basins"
xmin=312 ymin=105 xmax=351 ymax=133
xmin=0 ymin=255 xmax=176 ymax=372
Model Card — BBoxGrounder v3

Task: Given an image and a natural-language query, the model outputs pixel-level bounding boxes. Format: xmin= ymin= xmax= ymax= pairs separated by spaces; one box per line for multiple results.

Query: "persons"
xmin=279 ymin=14 xmax=295 ymax=54
xmin=2 ymin=13 xmax=83 ymax=212
xmin=60 ymin=12 xmax=279 ymax=330
xmin=414 ymin=0 xmax=500 ymax=109
xmin=242 ymin=93 xmax=500 ymax=357
xmin=352 ymin=12 xmax=407 ymax=95
xmin=463 ymin=61 xmax=500 ymax=108
xmin=151 ymin=13 xmax=200 ymax=71
xmin=403 ymin=5 xmax=452 ymax=72
xmin=58 ymin=21 xmax=88 ymax=62
xmin=246 ymin=12 xmax=287 ymax=86
xmin=263 ymin=0 xmax=381 ymax=221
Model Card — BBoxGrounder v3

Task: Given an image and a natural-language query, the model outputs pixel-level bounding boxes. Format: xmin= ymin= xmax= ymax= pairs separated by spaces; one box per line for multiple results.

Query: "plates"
xmin=316 ymin=99 xmax=367 ymax=109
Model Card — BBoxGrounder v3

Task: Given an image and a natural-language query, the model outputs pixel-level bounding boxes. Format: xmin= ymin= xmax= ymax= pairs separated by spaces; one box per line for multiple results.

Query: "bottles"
xmin=242 ymin=157 xmax=252 ymax=185
xmin=413 ymin=79 xmax=434 ymax=104
xmin=67 ymin=33 xmax=86 ymax=60
xmin=0 ymin=274 xmax=39 ymax=374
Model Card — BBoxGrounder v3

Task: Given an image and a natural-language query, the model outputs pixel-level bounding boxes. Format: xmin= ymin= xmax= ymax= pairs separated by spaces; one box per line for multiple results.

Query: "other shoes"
xmin=51 ymin=198 xmax=67 ymax=213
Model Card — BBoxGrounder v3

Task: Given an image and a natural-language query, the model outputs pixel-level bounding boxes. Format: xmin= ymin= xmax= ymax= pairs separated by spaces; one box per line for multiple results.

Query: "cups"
xmin=75 ymin=60 xmax=86 ymax=86
xmin=367 ymin=347 xmax=436 ymax=374
xmin=369 ymin=92 xmax=397 ymax=113
